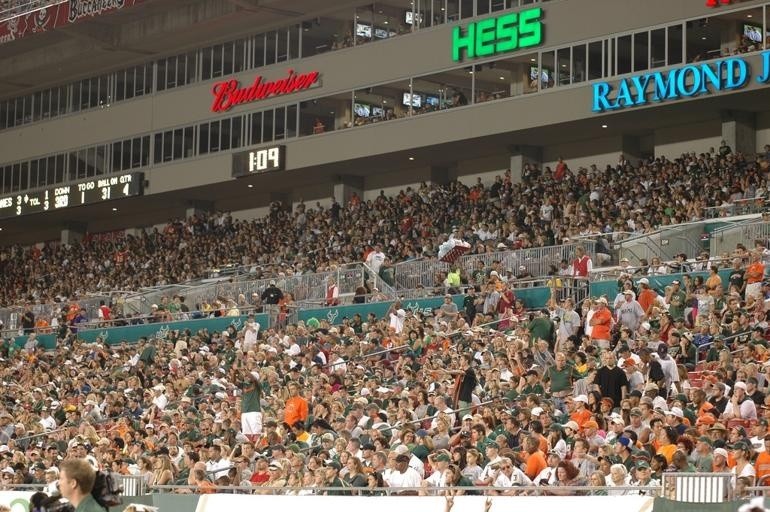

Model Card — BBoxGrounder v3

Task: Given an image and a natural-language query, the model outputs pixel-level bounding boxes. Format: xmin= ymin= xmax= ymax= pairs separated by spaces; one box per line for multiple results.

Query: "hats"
xmin=695 ymin=417 xmax=768 ymax=466
xmin=500 ymin=383 xmax=684 ymax=452
xmin=734 ymin=381 xmax=750 ymax=396
xmin=255 ymin=419 xmax=342 ymax=470
xmin=595 ymin=278 xmax=649 ymax=303
xmin=635 ymin=460 xmax=649 ymax=469
xmin=0 ymin=389 xmax=95 ymax=474
xmin=711 ymin=382 xmax=725 ymax=394
xmin=360 ymin=382 xmax=499 ymax=462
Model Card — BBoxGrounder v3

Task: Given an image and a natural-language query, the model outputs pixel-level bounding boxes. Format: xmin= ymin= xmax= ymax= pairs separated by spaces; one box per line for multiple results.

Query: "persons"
xmin=313 ymin=17 xmax=769 ymax=132
xmin=2 ymin=139 xmax=769 ymax=511
